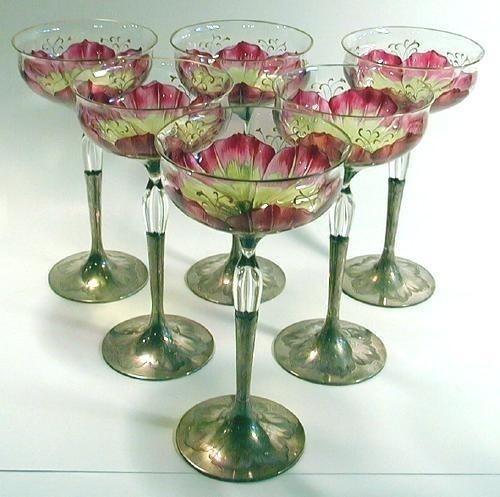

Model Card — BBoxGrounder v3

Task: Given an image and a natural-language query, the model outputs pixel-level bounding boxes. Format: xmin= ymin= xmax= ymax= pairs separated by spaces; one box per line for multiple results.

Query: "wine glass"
xmin=153 ymin=104 xmax=351 ymax=482
xmin=10 ymin=19 xmax=160 ymax=304
xmin=71 ymin=57 xmax=235 ymax=382
xmin=271 ymin=63 xmax=434 ymax=387
xmin=341 ymin=25 xmax=485 ymax=308
xmin=169 ymin=20 xmax=314 ymax=306
